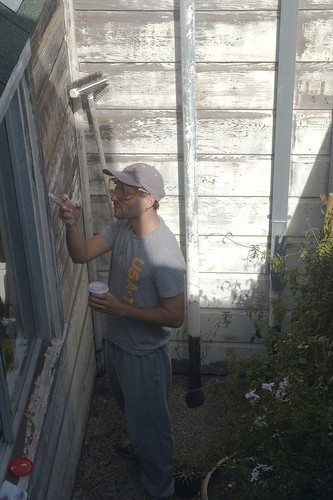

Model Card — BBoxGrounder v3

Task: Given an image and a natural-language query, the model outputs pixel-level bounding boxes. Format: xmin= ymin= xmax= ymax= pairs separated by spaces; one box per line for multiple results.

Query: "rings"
xmin=98 ymin=304 xmax=101 ymax=311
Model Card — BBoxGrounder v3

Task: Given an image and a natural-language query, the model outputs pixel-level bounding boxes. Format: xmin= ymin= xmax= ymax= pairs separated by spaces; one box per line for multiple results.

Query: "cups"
xmin=89 ymin=282 xmax=109 ymax=299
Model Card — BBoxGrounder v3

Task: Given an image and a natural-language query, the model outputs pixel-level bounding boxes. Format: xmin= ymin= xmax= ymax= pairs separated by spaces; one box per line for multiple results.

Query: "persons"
xmin=47 ymin=163 xmax=186 ymax=500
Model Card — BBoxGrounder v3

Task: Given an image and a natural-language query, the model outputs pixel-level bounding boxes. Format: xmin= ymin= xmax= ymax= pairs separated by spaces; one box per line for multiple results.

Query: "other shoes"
xmin=118 ymin=439 xmax=144 ymax=457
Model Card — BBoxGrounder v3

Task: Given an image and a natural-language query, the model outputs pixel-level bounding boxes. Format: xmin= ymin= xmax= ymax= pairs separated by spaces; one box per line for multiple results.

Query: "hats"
xmin=102 ymin=162 xmax=166 ymax=202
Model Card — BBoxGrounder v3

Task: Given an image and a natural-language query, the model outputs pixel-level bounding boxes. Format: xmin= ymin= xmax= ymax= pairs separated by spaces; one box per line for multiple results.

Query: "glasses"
xmin=108 ymin=178 xmax=149 ymax=201
